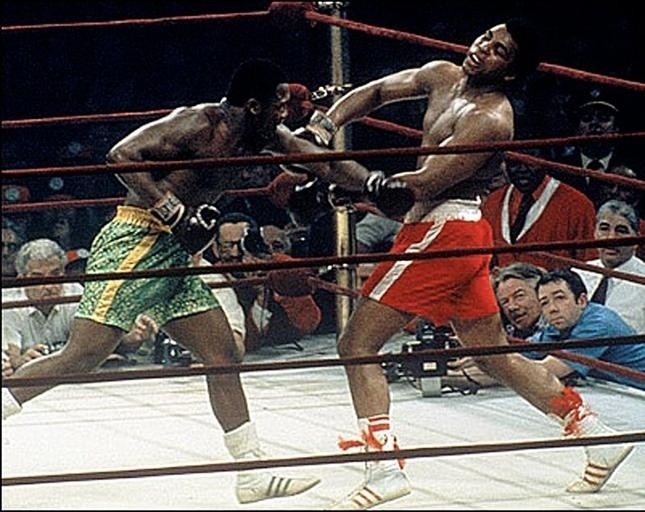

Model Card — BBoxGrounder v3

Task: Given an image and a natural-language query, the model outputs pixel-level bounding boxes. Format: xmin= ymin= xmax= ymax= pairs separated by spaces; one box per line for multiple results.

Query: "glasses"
xmin=214 ymin=239 xmax=240 ymax=248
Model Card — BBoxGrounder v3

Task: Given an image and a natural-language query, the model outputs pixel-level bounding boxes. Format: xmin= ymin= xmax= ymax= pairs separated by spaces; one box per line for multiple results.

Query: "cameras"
xmin=154 ymin=332 xmax=192 ymax=368
xmin=238 ymin=225 xmax=311 ymax=256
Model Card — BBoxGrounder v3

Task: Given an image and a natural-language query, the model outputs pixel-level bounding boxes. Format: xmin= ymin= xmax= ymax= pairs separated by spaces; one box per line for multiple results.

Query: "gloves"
xmin=277 ymin=109 xmax=340 ymax=178
xmin=328 ymin=169 xmax=415 ymax=215
xmin=148 ymin=190 xmax=223 ymax=255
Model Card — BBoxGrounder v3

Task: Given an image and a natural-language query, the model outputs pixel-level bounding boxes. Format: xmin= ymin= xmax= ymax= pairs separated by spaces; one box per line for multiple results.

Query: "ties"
xmin=590 ymin=276 xmax=609 ymax=306
xmin=587 ymin=160 xmax=603 ymax=200
xmin=510 ymin=194 xmax=534 ymax=244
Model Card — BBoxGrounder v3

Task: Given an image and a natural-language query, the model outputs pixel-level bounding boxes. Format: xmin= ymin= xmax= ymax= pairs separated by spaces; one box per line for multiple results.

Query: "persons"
xmin=0 ymin=59 xmax=416 ymax=503
xmin=280 ymin=21 xmax=634 ymax=509
xmin=0 ymin=40 xmax=645 ymax=389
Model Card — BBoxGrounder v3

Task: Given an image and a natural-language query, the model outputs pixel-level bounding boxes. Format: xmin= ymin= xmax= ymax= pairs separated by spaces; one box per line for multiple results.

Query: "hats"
xmin=3 ymin=184 xmax=31 ymax=204
xmin=59 ymin=142 xmax=91 ymax=157
xmin=573 ymin=86 xmax=615 ymax=112
xmin=40 ymin=176 xmax=78 ymax=197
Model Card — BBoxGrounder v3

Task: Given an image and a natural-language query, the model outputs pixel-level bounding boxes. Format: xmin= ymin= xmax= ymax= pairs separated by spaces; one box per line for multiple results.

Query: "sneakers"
xmin=323 ymin=469 xmax=412 ymax=510
xmin=566 ymin=432 xmax=635 ymax=493
xmin=234 ymin=471 xmax=321 ymax=503
